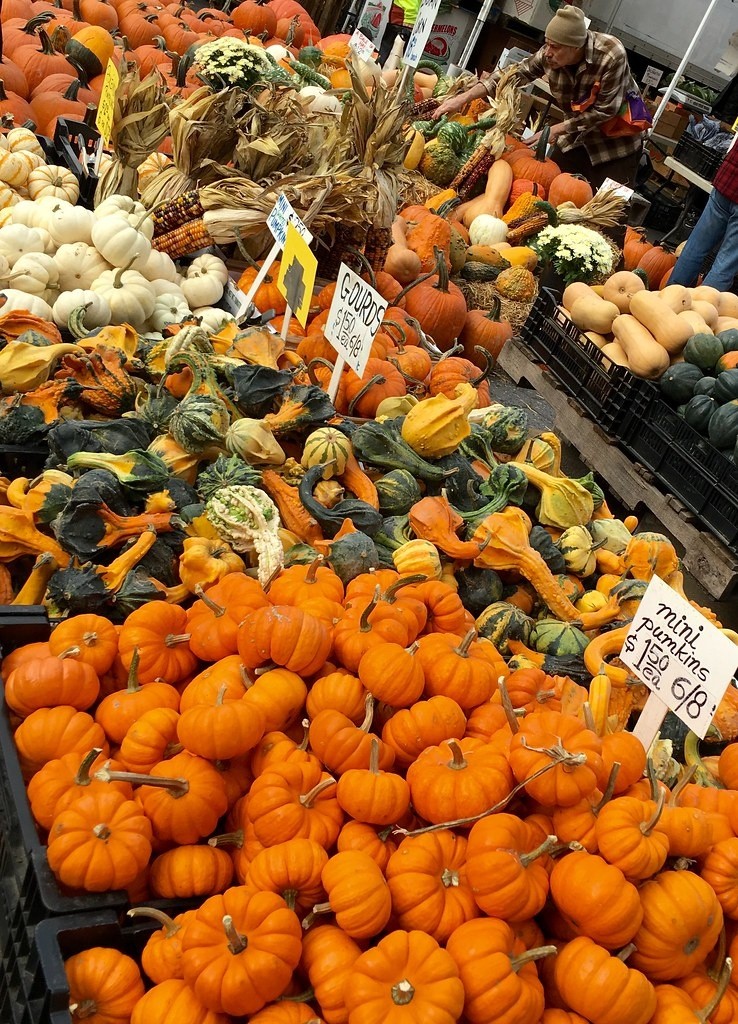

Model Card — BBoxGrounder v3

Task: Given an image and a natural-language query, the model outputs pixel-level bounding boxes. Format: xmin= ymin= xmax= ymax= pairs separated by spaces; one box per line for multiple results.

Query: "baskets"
xmin=673 ymin=130 xmax=724 ymax=181
xmin=639 ymin=184 xmax=682 ymax=234
xmin=615 ymin=380 xmax=738 ymax=558
xmin=521 ymin=287 xmax=658 ymax=436
xmin=0 ymin=442 xmax=231 ymax=1024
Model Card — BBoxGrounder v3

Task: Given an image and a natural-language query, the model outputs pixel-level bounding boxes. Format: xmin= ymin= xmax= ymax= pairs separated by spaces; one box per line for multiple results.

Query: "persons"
xmin=433 ymin=5 xmax=654 ymax=273
xmin=664 ymin=137 xmax=737 ymax=296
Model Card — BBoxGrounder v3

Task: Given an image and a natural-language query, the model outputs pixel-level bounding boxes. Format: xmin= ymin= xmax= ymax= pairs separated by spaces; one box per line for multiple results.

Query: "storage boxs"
xmin=0 ymin=13 xmax=738 ymax=1022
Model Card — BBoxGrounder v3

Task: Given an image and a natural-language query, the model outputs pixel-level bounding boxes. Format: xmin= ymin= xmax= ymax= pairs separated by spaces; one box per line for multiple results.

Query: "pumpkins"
xmin=0 ymin=0 xmax=737 ymax=1024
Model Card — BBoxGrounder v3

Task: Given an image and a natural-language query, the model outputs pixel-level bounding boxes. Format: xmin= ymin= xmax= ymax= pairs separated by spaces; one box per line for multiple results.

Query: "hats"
xmin=545 ymin=4 xmax=587 ymax=47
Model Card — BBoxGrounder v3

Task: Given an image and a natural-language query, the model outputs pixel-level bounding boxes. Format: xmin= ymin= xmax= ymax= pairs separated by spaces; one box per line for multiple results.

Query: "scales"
xmin=655 ymin=86 xmax=713 ymax=116
